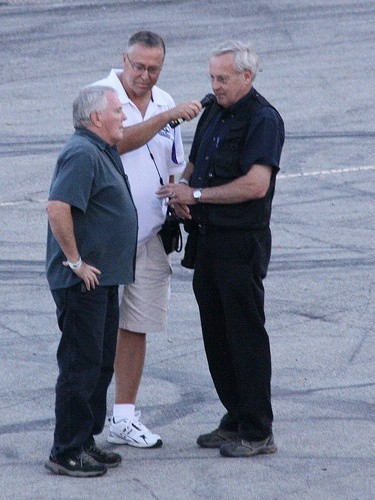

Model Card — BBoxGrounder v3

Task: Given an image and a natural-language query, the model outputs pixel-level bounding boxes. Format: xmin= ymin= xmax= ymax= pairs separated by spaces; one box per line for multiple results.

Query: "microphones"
xmin=169 ymin=93 xmax=217 ymax=127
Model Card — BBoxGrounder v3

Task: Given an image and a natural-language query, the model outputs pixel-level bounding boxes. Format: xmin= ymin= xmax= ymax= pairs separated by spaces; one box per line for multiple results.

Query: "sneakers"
xmin=82 ymin=436 xmax=122 ymax=469
xmin=220 ymin=434 xmax=277 ymax=457
xmin=107 ymin=410 xmax=163 ymax=449
xmin=45 ymin=447 xmax=108 ymax=478
xmin=197 ymin=427 xmax=238 ymax=449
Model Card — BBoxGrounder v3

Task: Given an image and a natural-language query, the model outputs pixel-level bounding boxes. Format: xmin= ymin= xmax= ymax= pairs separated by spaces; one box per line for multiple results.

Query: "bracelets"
xmin=179 ymin=178 xmax=189 ymax=186
xmin=62 ymin=256 xmax=83 ymax=269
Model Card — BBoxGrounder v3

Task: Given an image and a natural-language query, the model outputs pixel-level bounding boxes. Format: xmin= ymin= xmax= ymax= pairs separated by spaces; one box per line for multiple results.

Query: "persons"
xmin=81 ymin=31 xmax=204 ymax=448
xmin=157 ymin=41 xmax=286 ymax=456
xmin=42 ymin=85 xmax=140 ymax=479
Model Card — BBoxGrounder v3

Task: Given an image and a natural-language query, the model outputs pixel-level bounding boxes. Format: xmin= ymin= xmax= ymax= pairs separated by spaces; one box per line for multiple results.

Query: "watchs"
xmin=193 ymin=187 xmax=203 ymax=203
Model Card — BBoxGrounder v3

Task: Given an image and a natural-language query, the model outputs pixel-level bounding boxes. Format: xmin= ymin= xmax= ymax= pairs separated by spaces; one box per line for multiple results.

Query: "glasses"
xmin=127 ymin=54 xmax=162 ymax=75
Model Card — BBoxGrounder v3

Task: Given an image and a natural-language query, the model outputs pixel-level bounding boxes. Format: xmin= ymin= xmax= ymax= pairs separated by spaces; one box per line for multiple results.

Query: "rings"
xmin=168 ymin=192 xmax=175 ymax=198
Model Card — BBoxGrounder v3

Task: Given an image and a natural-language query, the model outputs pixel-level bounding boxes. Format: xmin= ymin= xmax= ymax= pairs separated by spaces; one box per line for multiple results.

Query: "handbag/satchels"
xmin=162 ymin=216 xmax=184 ymax=255
